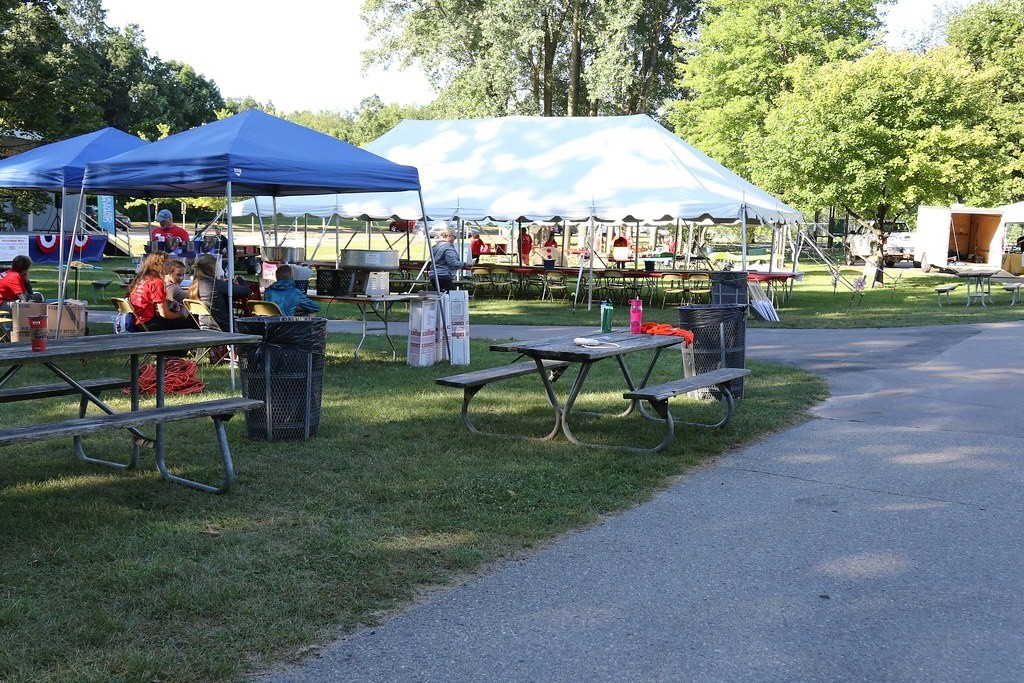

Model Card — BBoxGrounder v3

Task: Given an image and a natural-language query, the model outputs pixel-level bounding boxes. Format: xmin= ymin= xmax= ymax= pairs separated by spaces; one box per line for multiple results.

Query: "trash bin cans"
xmin=676 ymin=304 xmax=749 ymax=400
xmin=234 ymin=316 xmax=328 ymax=441
xmin=709 ymin=272 xmax=748 ymax=303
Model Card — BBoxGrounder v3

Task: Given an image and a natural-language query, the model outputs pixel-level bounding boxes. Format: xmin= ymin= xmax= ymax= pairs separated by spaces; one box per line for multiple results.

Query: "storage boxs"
xmin=46 ymin=301 xmax=87 ymax=340
xmin=10 ymin=330 xmax=42 ymax=343
xmin=407 ymin=288 xmax=469 ymax=368
xmin=13 ymin=301 xmax=44 ymax=332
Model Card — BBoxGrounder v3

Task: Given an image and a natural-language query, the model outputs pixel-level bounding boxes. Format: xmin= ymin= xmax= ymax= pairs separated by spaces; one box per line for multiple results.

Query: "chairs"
xmin=247 ymin=300 xmax=282 ymax=318
xmin=0 ymin=310 xmax=13 ymax=342
xmin=112 ymin=296 xmax=190 ymax=362
xmin=183 ymin=298 xmax=239 ymax=371
xmin=470 ymin=265 xmax=712 ymax=300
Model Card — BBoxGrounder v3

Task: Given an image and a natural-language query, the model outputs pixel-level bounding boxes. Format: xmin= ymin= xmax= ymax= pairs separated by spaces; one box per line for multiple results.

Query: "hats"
xmin=215 ymin=226 xmax=221 ymax=230
xmin=195 ymin=227 xmax=202 ymax=232
xmin=153 ymin=209 xmax=172 ymax=222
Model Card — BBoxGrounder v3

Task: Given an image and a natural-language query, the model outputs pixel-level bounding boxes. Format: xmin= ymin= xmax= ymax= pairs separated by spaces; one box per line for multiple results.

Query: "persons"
xmin=127 ymin=209 xmax=252 ymax=334
xmin=666 ymin=235 xmax=675 ymax=254
xmin=264 ymin=264 xmax=321 ymax=318
xmin=428 ymin=227 xmax=474 ymax=293
xmin=543 ymin=234 xmax=557 ymax=248
xmin=517 ymin=227 xmax=533 ymax=266
xmin=614 ymin=234 xmax=627 ymax=269
xmin=0 ymin=255 xmax=45 ymax=311
xmin=471 ymin=234 xmax=485 ymax=264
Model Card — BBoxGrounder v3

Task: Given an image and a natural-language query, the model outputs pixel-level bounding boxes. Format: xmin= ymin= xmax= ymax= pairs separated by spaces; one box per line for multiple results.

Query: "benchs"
xmin=0 ymin=396 xmax=262 ymax=446
xmin=1002 ymin=282 xmax=1022 ymax=299
xmin=934 ymin=283 xmax=959 ymax=303
xmin=0 ymin=377 xmax=133 ymax=404
xmin=436 ymin=358 xmax=573 ymax=389
xmin=625 ymin=362 xmax=750 ymax=402
xmin=92 ymin=280 xmax=114 ymax=287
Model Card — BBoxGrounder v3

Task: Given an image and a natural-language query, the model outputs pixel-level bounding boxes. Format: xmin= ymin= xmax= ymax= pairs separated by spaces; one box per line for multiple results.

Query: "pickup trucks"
xmin=844 ymin=220 xmax=915 ymax=266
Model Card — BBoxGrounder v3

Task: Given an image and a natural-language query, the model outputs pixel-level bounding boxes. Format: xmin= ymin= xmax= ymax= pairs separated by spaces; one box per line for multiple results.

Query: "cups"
xmin=28 ymin=316 xmax=47 ymax=351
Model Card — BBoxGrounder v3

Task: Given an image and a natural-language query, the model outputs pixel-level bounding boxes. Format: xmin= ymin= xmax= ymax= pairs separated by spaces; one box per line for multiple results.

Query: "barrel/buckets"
xmin=543 ymin=259 xmax=555 ymax=270
xmin=644 ymin=261 xmax=655 ymax=271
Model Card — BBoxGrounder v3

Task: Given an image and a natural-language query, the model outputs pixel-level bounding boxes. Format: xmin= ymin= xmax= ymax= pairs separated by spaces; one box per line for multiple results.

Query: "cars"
xmin=85 ymin=206 xmax=131 ymax=231
xmin=388 ymin=220 xmax=416 ymax=232
xmin=412 ymin=221 xmax=481 ymax=239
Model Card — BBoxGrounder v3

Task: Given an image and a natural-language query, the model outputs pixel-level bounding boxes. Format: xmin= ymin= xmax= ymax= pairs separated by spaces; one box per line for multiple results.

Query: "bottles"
xmin=628 ymin=299 xmax=642 ymax=334
xmin=602 ymin=297 xmax=614 ymax=332
xmin=601 ymin=301 xmax=606 ymax=332
xmin=176 ymin=237 xmax=182 ymax=248
xmin=167 ymin=236 xmax=172 ymax=246
xmin=182 ymin=240 xmax=187 ymax=250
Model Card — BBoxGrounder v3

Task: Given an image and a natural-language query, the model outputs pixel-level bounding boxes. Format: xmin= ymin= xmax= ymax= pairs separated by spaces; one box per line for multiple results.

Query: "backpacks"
xmin=112 ymin=284 xmax=152 ymax=334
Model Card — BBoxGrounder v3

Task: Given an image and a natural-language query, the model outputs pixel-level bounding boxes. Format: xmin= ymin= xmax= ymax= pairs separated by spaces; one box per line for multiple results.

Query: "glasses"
xmin=447 ymin=234 xmax=456 ymax=239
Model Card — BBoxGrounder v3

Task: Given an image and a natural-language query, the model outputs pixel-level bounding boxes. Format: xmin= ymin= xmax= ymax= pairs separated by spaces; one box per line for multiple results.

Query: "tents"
xmin=54 ymin=112 xmax=452 ymax=370
xmin=1 ymin=127 xmax=268 ymax=339
xmin=193 ymin=115 xmax=908 ymax=311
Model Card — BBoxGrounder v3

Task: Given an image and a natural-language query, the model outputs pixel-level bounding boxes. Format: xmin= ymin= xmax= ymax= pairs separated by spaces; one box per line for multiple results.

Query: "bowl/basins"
xmin=204 ymin=236 xmax=214 ymax=241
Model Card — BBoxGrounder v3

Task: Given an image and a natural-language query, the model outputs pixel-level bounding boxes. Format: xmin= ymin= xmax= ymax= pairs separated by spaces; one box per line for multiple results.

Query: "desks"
xmin=953 ymin=268 xmax=1002 ymax=305
xmin=394 ymin=261 xmax=798 ymax=311
xmin=488 ymin=327 xmax=684 ymax=445
xmin=112 ymin=265 xmax=140 ymax=299
xmin=315 ymin=293 xmax=420 ymax=363
xmin=0 ymin=328 xmax=262 ymax=491
